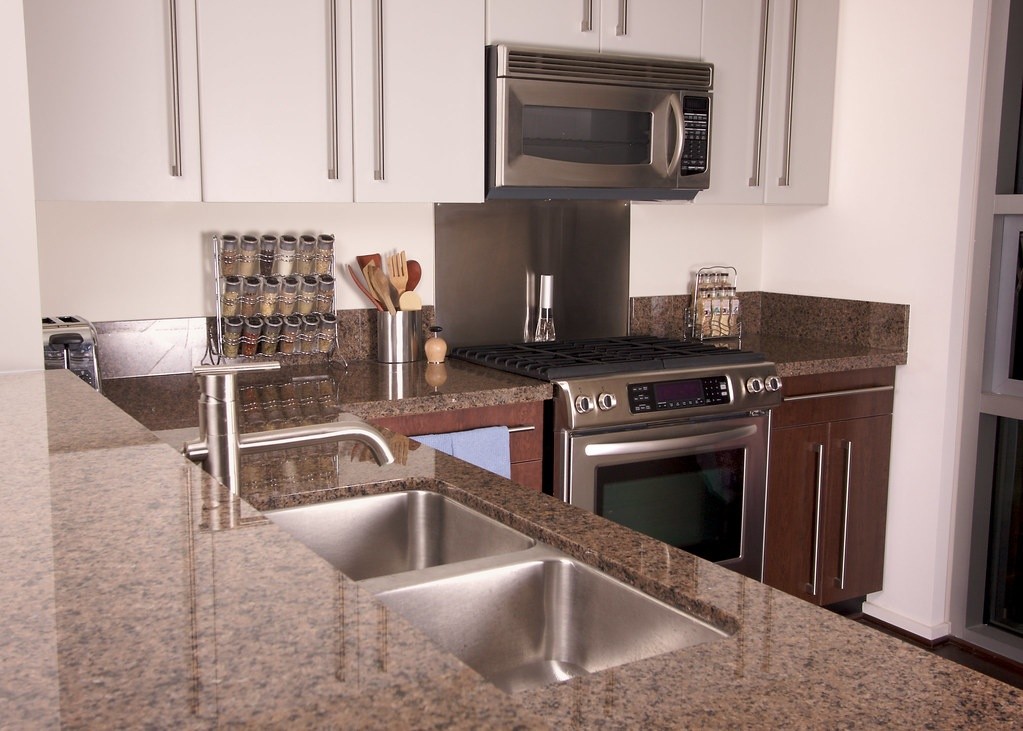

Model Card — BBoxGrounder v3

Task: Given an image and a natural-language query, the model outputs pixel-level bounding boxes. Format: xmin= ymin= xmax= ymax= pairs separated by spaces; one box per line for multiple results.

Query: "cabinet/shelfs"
xmin=366 ymin=398 xmax=543 ymax=493
xmin=485 ymin=0 xmax=703 ymax=61
xmin=22 ymin=0 xmax=203 ymax=199
xmin=194 ymin=1 xmax=486 ymax=204
xmin=763 ymin=366 xmax=897 ymax=605
xmin=701 ymin=0 xmax=840 ymax=207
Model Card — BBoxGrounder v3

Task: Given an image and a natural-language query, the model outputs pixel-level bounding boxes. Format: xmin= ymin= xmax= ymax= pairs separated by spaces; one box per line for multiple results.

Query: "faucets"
xmin=181 ymin=362 xmax=397 ymax=498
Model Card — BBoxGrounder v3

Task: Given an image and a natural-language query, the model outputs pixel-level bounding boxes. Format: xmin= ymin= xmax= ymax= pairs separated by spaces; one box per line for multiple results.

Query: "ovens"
xmin=483 ymin=41 xmax=714 ymax=200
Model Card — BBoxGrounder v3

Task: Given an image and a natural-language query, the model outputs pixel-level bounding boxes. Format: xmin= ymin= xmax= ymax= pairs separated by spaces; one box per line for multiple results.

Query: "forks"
xmin=387 ymin=250 xmax=409 ymax=312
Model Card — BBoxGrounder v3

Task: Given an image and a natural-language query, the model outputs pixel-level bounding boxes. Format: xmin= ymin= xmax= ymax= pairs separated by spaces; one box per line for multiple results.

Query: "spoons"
xmin=406 ymin=260 xmax=422 ymax=293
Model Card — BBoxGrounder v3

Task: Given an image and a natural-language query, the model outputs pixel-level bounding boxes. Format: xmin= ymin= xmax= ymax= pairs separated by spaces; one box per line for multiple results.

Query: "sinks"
xmin=373 ymin=558 xmax=730 ymax=699
xmin=257 ymin=490 xmax=538 ymax=583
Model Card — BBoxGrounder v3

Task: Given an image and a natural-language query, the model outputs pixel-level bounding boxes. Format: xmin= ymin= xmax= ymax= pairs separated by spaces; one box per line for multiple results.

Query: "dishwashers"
xmin=552 ymin=360 xmax=783 ymax=574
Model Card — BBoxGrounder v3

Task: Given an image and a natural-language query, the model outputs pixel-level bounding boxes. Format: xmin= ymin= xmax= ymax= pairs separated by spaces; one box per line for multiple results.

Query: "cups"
xmin=375 ymin=310 xmax=422 ymax=362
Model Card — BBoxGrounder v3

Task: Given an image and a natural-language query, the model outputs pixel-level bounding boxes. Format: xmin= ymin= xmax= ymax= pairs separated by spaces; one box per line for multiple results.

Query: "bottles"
xmin=238 ymin=375 xmax=341 ymax=496
xmin=426 ymin=326 xmax=447 ymax=364
xmin=218 ymin=234 xmax=334 ymax=355
xmin=425 ymin=364 xmax=448 ymax=396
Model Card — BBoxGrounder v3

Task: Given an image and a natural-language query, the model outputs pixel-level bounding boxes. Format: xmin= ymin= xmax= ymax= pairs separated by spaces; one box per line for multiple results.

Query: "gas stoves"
xmin=452 ymin=334 xmax=770 ymax=380
